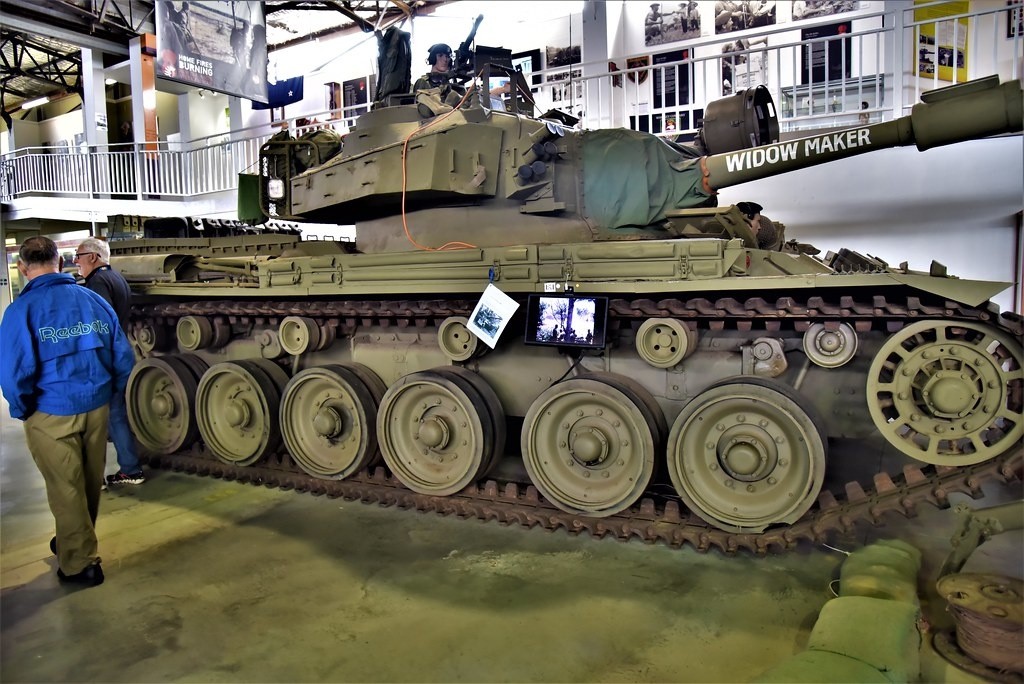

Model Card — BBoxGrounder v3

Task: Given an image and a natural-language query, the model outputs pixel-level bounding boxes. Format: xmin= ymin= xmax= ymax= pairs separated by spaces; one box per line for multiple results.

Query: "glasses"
xmin=76 ymin=252 xmax=101 ymax=259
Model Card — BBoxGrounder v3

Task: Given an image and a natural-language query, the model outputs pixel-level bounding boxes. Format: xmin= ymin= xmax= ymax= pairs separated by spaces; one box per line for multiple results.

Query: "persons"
xmin=230 ymin=16 xmax=266 ymax=94
xmin=570 ymin=330 xmax=577 ymax=342
xmin=553 ymin=325 xmax=558 ymax=339
xmin=1 ymin=236 xmax=135 ymax=592
xmin=558 ymin=329 xmax=565 ymax=340
xmin=586 ymin=329 xmax=593 ymax=343
xmin=715 ymin=0 xmax=776 ymax=34
xmin=157 ymin=1 xmax=205 ymax=57
xmin=645 ymin=1 xmax=701 ymax=44
xmin=721 ymin=39 xmax=752 ymax=91
xmin=75 ymin=238 xmax=148 ymax=485
xmin=793 ymin=0 xmax=856 ymax=20
xmin=736 ymin=201 xmax=763 ymax=237
xmin=414 ymin=43 xmax=453 ymax=93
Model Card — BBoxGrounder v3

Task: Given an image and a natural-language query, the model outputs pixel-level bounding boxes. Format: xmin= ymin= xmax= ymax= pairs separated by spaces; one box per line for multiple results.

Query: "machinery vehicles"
xmin=76 ymin=61 xmax=1024 ymax=558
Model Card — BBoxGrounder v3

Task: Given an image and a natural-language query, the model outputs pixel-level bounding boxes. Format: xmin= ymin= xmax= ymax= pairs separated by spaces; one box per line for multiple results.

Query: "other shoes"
xmin=50 ymin=535 xmax=57 ymax=555
xmin=58 ymin=563 xmax=104 ymax=586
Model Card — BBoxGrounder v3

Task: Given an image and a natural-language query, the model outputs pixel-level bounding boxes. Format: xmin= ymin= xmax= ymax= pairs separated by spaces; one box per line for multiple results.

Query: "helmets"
xmin=425 ymin=44 xmax=453 ymax=65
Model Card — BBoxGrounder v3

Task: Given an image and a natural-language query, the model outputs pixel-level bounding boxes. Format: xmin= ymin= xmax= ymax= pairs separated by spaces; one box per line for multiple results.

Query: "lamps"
xmin=22 ymin=97 xmax=50 ymax=110
xmin=105 ymin=79 xmax=118 ymax=86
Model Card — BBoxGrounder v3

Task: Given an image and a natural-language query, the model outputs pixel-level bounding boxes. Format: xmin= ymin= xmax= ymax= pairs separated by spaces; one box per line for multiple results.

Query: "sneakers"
xmin=106 ymin=471 xmax=146 ymax=485
xmin=100 ymin=478 xmax=107 ymax=491
